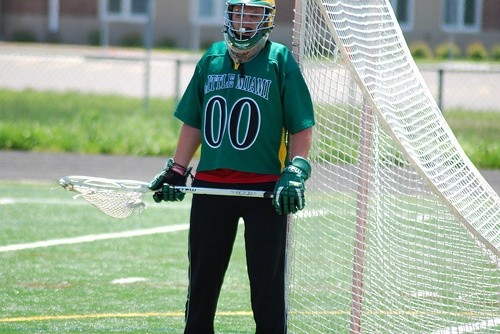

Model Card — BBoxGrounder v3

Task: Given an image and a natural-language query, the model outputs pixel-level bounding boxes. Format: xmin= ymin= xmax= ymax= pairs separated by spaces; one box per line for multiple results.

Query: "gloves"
xmin=272 ymin=155 xmax=312 ymax=215
xmin=149 ymin=158 xmax=193 ymax=203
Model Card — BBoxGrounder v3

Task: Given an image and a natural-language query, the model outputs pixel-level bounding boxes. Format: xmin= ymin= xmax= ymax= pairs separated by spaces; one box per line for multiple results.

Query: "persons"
xmin=148 ymin=0 xmax=316 ymax=334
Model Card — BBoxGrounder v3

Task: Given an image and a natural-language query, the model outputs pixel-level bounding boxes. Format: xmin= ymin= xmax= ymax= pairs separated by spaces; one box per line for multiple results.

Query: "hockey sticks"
xmin=59 ymin=174 xmax=274 ymax=220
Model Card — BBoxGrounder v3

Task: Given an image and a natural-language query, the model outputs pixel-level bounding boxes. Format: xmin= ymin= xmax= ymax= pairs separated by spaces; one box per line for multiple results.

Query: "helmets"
xmin=223 ymin=0 xmax=276 ymax=49
